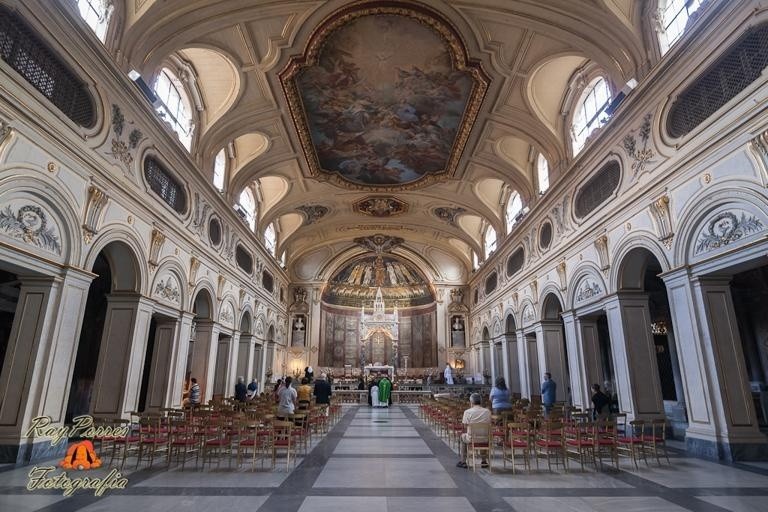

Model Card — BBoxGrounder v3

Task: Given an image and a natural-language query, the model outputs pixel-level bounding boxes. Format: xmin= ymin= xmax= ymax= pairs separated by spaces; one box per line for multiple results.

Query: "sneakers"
xmin=456 ymin=461 xmax=467 ymax=468
xmin=481 ymin=461 xmax=489 ymax=467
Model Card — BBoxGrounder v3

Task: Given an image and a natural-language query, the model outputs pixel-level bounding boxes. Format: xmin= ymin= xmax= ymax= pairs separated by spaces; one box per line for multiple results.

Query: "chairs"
xmin=417 ymin=391 xmax=671 ymax=475
xmin=88 ymin=389 xmax=343 ymax=473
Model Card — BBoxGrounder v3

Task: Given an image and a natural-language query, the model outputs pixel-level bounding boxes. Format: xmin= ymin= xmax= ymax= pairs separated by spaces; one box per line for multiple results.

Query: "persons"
xmin=183 ymin=366 xmax=332 ymax=441
xmin=456 ymin=393 xmax=492 ymax=468
xmin=444 ymin=362 xmax=454 ymax=385
xmin=568 ymin=380 xmax=619 ymax=428
xmin=489 ymin=376 xmax=514 ymax=446
xmin=541 ymin=373 xmax=557 ymax=416
